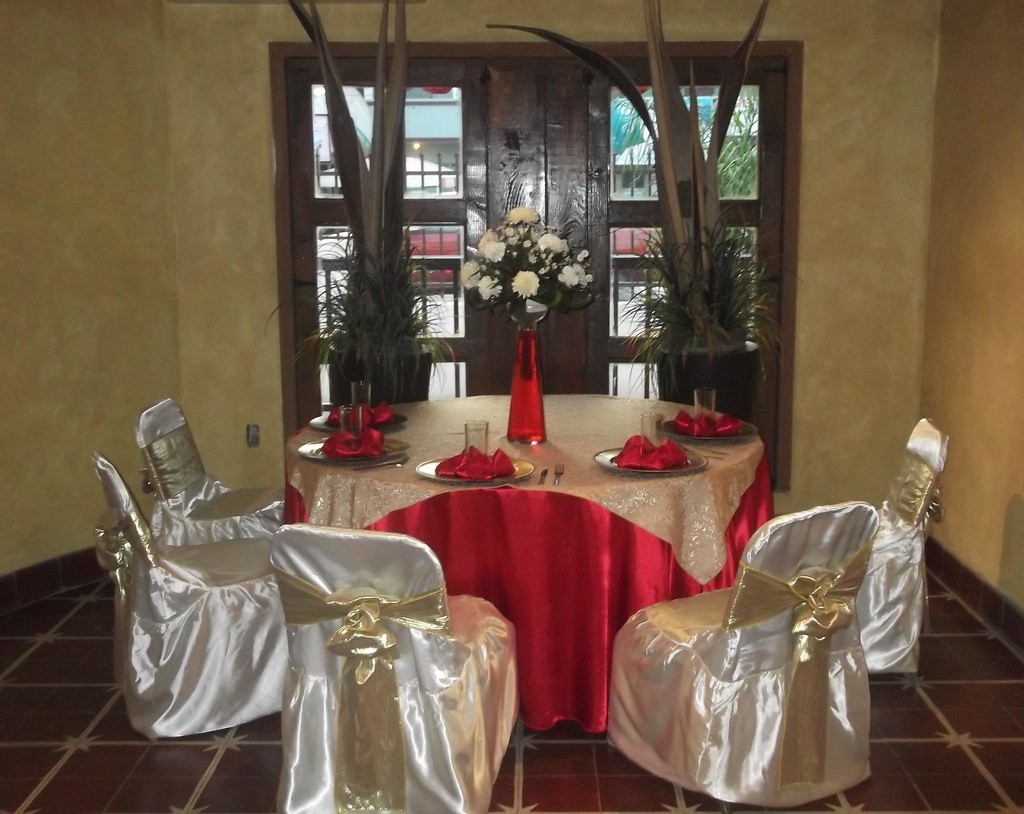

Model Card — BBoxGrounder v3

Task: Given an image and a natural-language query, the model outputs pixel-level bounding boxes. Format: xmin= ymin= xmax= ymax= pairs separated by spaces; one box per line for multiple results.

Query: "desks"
xmin=284 ymin=394 xmax=774 ymax=733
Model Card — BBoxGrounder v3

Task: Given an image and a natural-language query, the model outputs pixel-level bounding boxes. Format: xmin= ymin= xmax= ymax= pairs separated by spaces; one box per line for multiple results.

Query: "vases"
xmin=505 ymin=301 xmax=549 ymax=445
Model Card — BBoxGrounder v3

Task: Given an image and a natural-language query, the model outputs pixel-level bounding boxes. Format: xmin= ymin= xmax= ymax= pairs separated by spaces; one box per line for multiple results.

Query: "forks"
xmin=554 ymin=464 xmax=565 ymax=488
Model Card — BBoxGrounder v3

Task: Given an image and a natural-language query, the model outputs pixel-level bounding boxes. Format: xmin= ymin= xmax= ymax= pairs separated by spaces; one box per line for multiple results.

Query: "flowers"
xmin=458 ymin=211 xmax=594 ymax=321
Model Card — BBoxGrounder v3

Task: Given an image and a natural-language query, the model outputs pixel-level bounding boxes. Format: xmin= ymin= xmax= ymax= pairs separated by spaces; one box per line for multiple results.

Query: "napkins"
xmin=672 ymin=408 xmax=742 ymax=435
xmin=610 ymin=433 xmax=687 ymax=471
xmin=322 ymin=426 xmax=385 ymax=458
xmin=326 ymin=401 xmax=393 ymax=426
xmin=435 ymin=445 xmax=516 ymax=482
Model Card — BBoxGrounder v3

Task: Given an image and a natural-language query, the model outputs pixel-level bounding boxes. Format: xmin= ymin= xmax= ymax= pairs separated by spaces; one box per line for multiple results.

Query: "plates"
xmin=308 ymin=414 xmax=408 ymax=429
xmin=594 ymin=448 xmax=708 ymax=475
xmin=663 ymin=420 xmax=758 ymax=441
xmin=297 ymin=438 xmax=411 ymax=463
xmin=416 ymin=458 xmax=536 ymax=482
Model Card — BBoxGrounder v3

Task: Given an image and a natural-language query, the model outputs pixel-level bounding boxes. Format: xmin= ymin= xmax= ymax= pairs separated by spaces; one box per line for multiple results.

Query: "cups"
xmin=351 ymin=381 xmax=371 ymax=408
xmin=640 ymin=413 xmax=664 ymax=453
xmin=339 ymin=405 xmax=362 ymax=450
xmin=464 ymin=420 xmax=488 ymax=458
xmin=694 ymin=388 xmax=716 ymax=419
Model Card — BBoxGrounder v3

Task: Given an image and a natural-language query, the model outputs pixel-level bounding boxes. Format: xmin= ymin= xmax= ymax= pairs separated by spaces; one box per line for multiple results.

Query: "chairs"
xmin=269 ymin=525 xmax=519 ymax=814
xmin=610 ymin=500 xmax=880 ymax=808
xmin=90 ymin=451 xmax=287 ymax=739
xmin=138 ymin=397 xmax=286 ymax=546
xmin=854 ymin=415 xmax=948 ymax=673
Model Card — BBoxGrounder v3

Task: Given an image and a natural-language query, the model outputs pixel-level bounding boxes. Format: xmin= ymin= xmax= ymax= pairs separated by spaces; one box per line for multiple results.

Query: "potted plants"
xmin=484 ymin=1 xmax=781 ymax=423
xmin=264 ymin=1 xmax=457 ymax=403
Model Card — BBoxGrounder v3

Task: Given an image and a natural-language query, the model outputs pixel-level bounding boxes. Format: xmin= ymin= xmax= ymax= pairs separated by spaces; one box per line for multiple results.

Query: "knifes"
xmin=538 ymin=469 xmax=549 ymax=486
